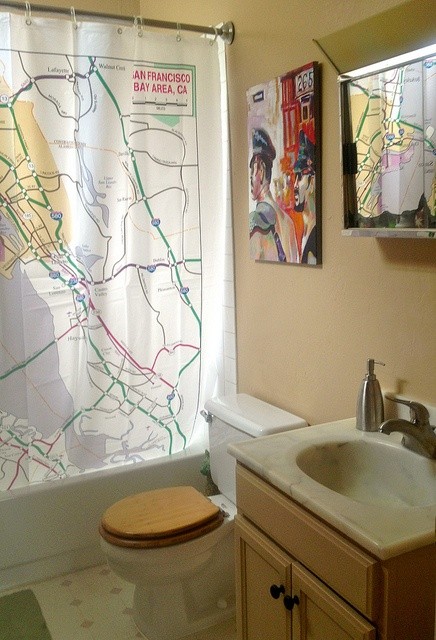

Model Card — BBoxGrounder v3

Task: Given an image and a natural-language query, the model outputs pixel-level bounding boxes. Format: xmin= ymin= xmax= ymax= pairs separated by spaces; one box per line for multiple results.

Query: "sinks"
xmin=292 ymin=435 xmax=436 ymax=513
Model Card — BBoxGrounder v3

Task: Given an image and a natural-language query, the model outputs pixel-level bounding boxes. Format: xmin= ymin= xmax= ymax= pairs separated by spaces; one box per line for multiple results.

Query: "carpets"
xmin=0 ymin=589 xmax=53 ymax=640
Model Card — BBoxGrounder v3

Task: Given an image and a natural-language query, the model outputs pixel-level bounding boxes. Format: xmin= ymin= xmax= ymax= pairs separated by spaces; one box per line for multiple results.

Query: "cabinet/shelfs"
xmin=234 ymin=462 xmax=378 ymax=640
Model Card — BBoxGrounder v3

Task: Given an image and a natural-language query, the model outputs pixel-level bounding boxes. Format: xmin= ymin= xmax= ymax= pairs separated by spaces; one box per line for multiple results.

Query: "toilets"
xmin=97 ymin=391 xmax=308 ymax=637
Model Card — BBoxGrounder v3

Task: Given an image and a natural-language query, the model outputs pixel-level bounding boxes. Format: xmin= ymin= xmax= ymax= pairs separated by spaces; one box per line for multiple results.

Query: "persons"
xmin=289 ymin=128 xmax=317 ymax=266
xmin=247 ymin=127 xmax=300 ymax=263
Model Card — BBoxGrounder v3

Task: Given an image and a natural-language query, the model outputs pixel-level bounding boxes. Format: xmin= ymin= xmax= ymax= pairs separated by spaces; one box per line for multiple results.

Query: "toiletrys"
xmin=353 ymin=358 xmax=388 ymax=431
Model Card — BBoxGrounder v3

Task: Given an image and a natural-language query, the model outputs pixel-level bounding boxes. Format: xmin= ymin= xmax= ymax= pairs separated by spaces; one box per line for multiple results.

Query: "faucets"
xmin=377 ymin=392 xmax=435 ymax=459
